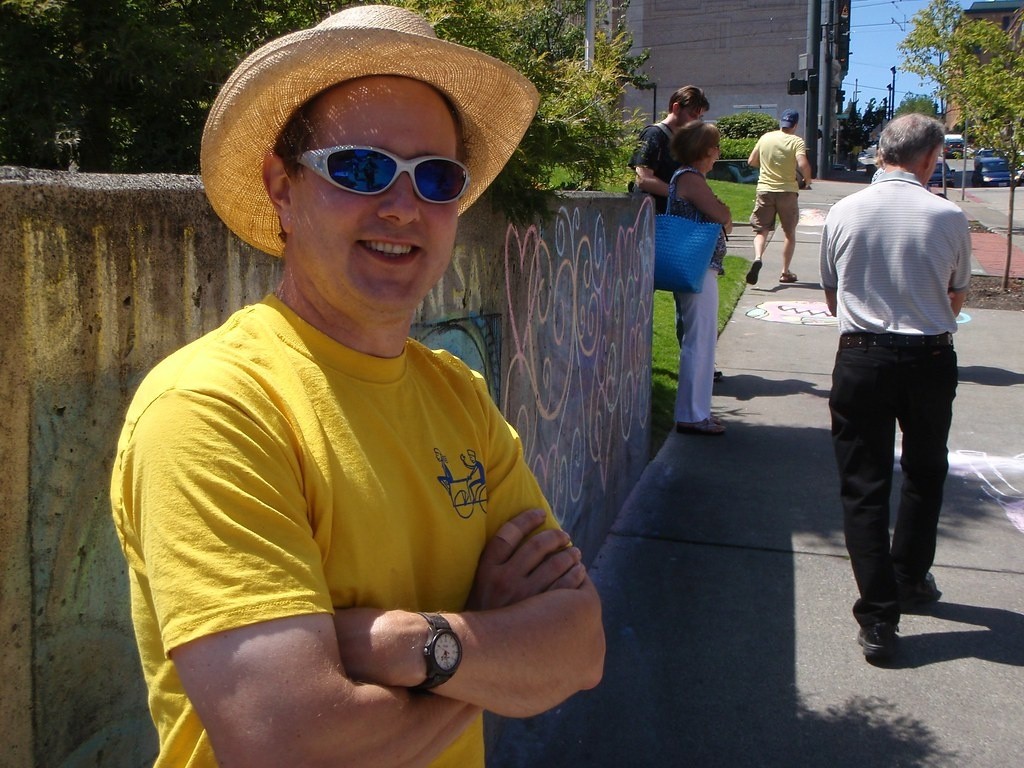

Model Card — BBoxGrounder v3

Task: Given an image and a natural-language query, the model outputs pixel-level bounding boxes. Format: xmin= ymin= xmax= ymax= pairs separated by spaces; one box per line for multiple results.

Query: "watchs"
xmin=409 ymin=611 xmax=463 ymax=693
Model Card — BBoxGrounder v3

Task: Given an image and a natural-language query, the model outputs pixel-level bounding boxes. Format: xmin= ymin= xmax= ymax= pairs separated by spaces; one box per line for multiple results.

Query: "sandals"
xmin=779 ymin=271 xmax=797 ymax=283
xmin=745 ymin=260 xmax=762 ymax=285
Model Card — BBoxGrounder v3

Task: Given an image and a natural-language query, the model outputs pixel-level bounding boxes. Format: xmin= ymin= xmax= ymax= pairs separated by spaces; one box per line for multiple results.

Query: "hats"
xmin=198 ymin=5 xmax=543 ymax=257
xmin=779 ymin=109 xmax=799 ymax=129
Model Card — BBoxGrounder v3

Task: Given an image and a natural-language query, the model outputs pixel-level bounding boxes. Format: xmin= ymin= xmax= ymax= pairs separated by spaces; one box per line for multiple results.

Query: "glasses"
xmin=289 ymin=144 xmax=471 ymax=205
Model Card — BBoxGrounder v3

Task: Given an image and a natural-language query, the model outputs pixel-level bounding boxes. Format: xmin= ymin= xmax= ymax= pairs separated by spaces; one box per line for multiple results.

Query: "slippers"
xmin=677 ymin=415 xmax=726 ymax=437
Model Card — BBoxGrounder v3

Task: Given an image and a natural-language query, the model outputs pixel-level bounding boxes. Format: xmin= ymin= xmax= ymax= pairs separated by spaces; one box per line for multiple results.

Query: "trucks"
xmin=942 ymin=133 xmax=964 ymax=159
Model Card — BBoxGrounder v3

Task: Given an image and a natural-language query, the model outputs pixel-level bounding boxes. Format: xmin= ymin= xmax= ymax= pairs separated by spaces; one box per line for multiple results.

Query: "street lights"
xmin=884 ymin=66 xmax=896 ymax=123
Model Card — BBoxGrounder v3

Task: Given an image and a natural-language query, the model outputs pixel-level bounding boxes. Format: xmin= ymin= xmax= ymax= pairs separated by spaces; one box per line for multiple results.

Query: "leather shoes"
xmin=856 ymin=621 xmax=899 ymax=658
xmin=893 ymin=572 xmax=940 ymax=612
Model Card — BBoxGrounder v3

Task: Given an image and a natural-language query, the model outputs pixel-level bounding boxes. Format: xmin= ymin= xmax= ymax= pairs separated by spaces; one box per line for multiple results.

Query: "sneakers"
xmin=713 ymin=371 xmax=722 ymax=382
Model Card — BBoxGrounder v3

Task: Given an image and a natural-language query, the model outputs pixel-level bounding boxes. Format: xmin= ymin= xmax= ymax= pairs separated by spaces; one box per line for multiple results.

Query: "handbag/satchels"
xmin=654 ymin=167 xmax=723 ymax=294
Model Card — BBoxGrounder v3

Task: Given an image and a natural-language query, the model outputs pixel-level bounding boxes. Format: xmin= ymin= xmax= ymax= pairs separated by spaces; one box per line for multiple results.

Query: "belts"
xmin=840 ymin=332 xmax=953 ymax=346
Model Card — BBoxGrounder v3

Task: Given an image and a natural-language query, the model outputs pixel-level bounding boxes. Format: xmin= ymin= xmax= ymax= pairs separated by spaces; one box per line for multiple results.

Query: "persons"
xmin=666 ymin=120 xmax=732 ymax=434
xmin=628 ymin=85 xmax=723 ymax=381
xmin=820 ymin=114 xmax=973 ymax=657
xmin=109 ymin=5 xmax=606 ymax=768
xmin=745 ymin=109 xmax=811 ymax=285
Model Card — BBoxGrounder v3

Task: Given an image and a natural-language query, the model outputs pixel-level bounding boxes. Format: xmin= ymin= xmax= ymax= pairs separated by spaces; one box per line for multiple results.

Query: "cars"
xmin=971 ymin=157 xmax=1021 ymax=187
xmin=925 ymin=161 xmax=956 ymax=189
xmin=970 ymin=147 xmax=1024 ymax=169
